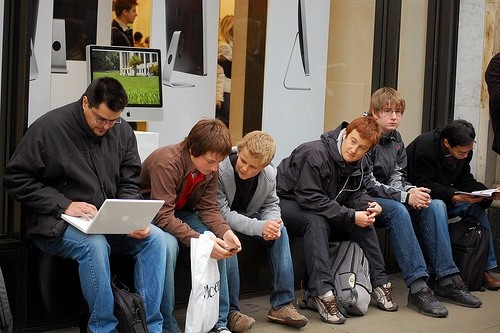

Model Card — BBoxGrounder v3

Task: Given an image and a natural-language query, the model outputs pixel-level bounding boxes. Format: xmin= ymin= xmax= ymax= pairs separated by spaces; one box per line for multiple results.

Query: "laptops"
xmin=163 ymin=31 xmax=196 ymax=88
xmin=61 ymin=198 xmax=165 ymax=234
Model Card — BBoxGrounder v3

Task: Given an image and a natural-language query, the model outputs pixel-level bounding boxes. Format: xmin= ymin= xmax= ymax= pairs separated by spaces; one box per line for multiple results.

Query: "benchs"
xmin=21 ymin=225 xmax=402 ymax=333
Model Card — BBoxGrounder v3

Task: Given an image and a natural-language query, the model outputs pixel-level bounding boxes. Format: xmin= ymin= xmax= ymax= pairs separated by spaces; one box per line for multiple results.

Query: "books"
xmin=454 ymin=187 xmax=500 ymax=203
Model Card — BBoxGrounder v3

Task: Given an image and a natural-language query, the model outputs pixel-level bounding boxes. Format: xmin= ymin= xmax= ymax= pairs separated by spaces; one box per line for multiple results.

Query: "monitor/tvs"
xmin=86 ymin=44 xmax=164 ymax=121
xmin=297 ymin=0 xmax=310 ymax=76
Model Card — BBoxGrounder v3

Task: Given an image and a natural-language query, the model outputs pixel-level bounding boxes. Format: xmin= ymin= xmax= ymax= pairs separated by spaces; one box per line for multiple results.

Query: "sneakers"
xmin=407 ymin=288 xmax=448 ymax=317
xmin=312 ymin=290 xmax=347 ymax=324
xmin=372 ymin=281 xmax=399 ymax=311
xmin=227 ymin=311 xmax=256 ymax=333
xmin=267 ymin=303 xmax=308 ymax=329
xmin=435 ymin=285 xmax=482 ymax=308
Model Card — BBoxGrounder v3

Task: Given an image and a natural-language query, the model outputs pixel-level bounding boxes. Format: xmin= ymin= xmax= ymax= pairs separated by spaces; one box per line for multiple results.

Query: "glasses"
xmin=87 ymin=99 xmax=122 ymax=125
xmin=453 ymin=148 xmax=474 ymax=155
xmin=377 ymin=108 xmax=403 ymax=115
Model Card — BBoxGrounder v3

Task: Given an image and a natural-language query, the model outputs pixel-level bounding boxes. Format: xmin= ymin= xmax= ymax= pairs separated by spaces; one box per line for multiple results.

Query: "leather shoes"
xmin=481 ymin=269 xmax=500 ymax=290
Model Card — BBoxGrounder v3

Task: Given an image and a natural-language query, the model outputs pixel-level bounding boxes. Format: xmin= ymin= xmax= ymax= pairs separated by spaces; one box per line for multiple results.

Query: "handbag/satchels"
xmin=184 ymin=230 xmax=220 ymax=333
xmin=79 ymin=273 xmax=148 ymax=333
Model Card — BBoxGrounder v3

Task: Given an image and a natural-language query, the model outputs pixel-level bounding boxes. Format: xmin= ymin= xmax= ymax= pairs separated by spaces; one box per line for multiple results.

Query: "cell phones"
xmin=228 ymin=245 xmax=240 ymax=253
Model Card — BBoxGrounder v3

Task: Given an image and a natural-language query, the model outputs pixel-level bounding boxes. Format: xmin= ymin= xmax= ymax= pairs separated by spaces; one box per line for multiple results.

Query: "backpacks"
xmin=328 ymin=240 xmax=372 ymax=317
xmin=450 ymin=223 xmax=491 ymax=292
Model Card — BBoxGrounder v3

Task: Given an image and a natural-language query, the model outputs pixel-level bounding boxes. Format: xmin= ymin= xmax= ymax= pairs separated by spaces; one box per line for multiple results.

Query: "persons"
xmin=275 ymin=116 xmax=399 ymax=325
xmin=358 ymin=87 xmax=484 ymax=320
xmin=2 ymin=74 xmax=168 ymax=333
xmin=111 ymin=1 xmax=235 ymax=132
xmin=215 ymin=129 xmax=308 ymax=333
xmin=484 ymin=51 xmax=500 ymax=156
xmin=405 ymin=115 xmax=499 ymax=292
xmin=135 ymin=117 xmax=244 ymax=333
